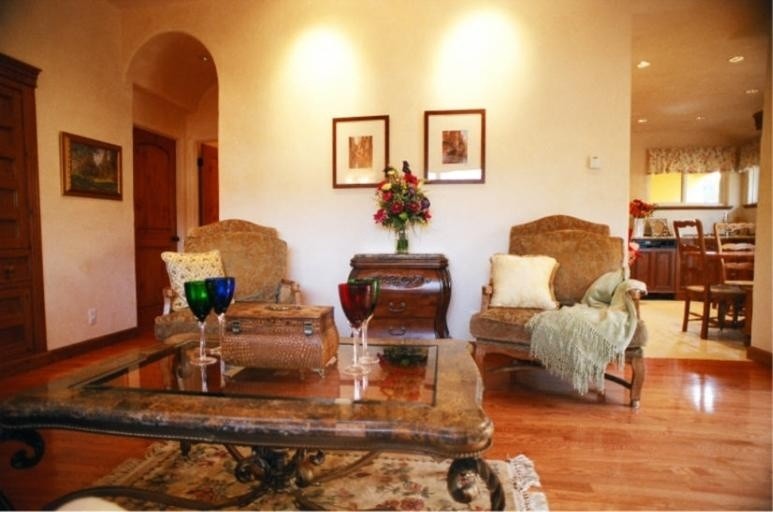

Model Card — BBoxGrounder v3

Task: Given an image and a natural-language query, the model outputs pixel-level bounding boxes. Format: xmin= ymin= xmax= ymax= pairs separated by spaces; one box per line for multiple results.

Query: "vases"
xmin=396 ymin=228 xmax=409 ymax=255
xmin=632 ymin=218 xmax=646 ymax=239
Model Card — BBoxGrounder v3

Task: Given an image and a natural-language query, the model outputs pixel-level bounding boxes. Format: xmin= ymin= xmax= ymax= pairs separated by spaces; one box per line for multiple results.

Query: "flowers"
xmin=373 ymin=160 xmax=431 ymax=230
xmin=631 ymin=198 xmax=658 ymax=219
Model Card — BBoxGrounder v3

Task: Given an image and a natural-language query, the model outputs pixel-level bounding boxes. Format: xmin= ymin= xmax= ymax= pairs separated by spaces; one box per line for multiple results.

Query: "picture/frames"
xmin=423 ymin=108 xmax=487 ymax=185
xmin=332 ymin=114 xmax=390 ymax=189
xmin=59 ymin=131 xmax=123 ymax=200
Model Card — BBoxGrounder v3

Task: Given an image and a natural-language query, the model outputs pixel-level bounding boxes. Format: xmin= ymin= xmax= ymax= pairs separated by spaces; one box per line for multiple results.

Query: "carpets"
xmin=88 ymin=441 xmax=549 ymax=511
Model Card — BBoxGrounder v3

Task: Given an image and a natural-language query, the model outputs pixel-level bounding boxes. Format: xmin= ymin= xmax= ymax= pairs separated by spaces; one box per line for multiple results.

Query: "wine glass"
xmin=338 ymin=282 xmax=375 ymax=404
xmin=182 ymin=278 xmax=219 ymax=370
xmin=205 ymin=275 xmax=239 ymax=378
xmin=348 ymin=278 xmax=381 ymax=367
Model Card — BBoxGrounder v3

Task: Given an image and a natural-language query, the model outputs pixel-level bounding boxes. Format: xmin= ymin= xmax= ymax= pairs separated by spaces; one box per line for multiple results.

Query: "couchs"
xmin=469 ymin=214 xmax=648 ymax=409
xmin=160 ymin=218 xmax=302 ymax=313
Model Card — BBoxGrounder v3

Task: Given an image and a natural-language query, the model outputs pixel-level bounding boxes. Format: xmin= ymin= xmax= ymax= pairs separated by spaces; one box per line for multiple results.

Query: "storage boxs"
xmin=221 ymin=299 xmax=340 ymax=378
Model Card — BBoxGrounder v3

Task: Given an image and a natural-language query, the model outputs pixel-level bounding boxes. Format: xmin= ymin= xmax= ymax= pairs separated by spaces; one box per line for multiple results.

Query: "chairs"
xmin=673 ymin=219 xmax=747 ymax=339
xmin=713 ymin=223 xmax=755 ymax=287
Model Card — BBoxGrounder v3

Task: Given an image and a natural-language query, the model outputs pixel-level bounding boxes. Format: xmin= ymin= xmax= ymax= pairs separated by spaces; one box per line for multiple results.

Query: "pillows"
xmin=161 ymin=248 xmax=235 ymax=312
xmin=202 ymin=232 xmax=288 ymax=302
xmin=489 ymin=253 xmax=562 ymax=308
xmin=515 ymin=229 xmax=624 ymax=305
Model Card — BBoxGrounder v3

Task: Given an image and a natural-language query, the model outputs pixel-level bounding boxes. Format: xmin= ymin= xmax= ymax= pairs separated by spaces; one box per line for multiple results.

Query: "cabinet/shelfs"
xmin=347 ymin=252 xmax=451 ymax=338
xmin=631 ymin=249 xmax=677 ymax=294
xmin=0 ymin=52 xmax=51 ymax=379
